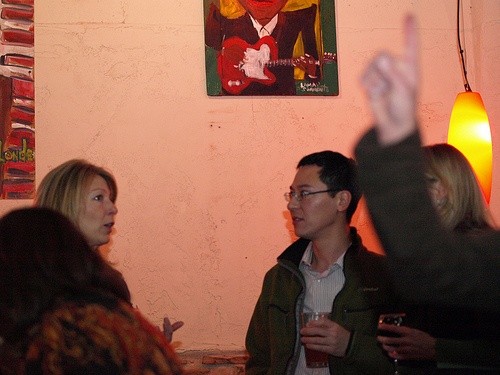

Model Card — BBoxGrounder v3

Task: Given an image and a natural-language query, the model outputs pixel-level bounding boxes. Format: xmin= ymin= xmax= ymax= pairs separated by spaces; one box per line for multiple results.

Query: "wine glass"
xmin=378 ymin=313 xmax=407 ymax=375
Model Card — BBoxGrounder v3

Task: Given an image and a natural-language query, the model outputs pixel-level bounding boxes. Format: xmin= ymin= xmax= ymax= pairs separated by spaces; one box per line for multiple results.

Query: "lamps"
xmin=446 ymin=0 xmax=494 ymax=204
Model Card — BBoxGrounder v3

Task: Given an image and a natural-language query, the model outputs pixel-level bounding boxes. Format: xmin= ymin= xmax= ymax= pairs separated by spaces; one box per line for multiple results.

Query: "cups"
xmin=302 ymin=311 xmax=329 ymax=368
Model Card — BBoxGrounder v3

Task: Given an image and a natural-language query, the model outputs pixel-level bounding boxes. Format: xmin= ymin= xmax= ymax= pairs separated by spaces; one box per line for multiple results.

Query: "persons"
xmin=0 ymin=206 xmax=130 ymax=338
xmin=36 ymin=159 xmax=184 ymax=344
xmin=353 ymin=13 xmax=500 ymax=375
xmin=377 ymin=144 xmax=490 ymax=375
xmin=244 ymin=151 xmax=401 ymax=375
xmin=0 ymin=292 xmax=184 ymax=375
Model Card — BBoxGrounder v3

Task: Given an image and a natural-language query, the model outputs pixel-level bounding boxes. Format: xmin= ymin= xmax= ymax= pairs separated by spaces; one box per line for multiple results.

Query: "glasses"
xmin=284 ymin=189 xmax=344 ymax=201
